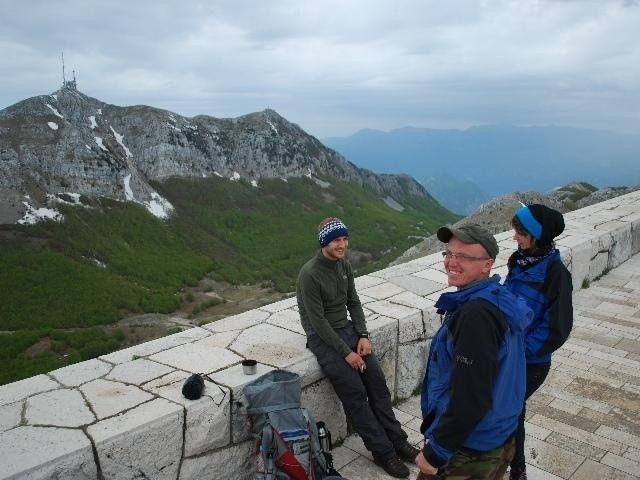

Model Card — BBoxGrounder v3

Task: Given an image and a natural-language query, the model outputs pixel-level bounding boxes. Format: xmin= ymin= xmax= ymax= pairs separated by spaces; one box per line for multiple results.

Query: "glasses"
xmin=441 ymin=250 xmax=484 ymax=261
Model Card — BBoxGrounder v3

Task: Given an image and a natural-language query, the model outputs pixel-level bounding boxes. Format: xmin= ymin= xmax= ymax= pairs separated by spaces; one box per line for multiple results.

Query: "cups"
xmin=241 ymin=359 xmax=259 ymax=375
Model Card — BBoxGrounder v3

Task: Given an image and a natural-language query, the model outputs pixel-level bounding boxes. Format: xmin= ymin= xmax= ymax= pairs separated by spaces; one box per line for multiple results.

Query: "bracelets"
xmin=360 ymin=334 xmax=370 ymax=340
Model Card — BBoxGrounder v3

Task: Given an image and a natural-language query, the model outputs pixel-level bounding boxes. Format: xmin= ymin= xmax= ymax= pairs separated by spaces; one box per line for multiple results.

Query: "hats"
xmin=515 ymin=199 xmax=566 ymax=247
xmin=431 ymin=218 xmax=501 ymax=259
xmin=316 ymin=215 xmax=351 ymax=248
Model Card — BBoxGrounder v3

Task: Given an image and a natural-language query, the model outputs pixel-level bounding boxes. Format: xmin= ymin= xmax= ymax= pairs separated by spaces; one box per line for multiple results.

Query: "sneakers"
xmin=398 ymin=441 xmax=422 ymax=463
xmin=372 ymin=453 xmax=411 ymax=479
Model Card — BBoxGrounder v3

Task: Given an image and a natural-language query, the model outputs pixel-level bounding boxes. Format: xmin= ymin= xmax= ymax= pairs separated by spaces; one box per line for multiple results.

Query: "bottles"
xmin=315 ymin=421 xmax=333 ymax=467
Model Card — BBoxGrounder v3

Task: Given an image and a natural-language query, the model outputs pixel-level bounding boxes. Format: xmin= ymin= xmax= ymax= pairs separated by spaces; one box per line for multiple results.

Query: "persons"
xmin=504 ymin=204 xmax=573 ymax=480
xmin=414 ymin=221 xmax=535 ymax=480
xmin=295 ymin=216 xmax=424 ymax=479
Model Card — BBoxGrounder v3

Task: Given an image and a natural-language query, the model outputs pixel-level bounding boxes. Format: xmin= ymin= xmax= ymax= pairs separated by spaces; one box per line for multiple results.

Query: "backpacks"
xmin=243 ymin=368 xmax=348 ymax=480
